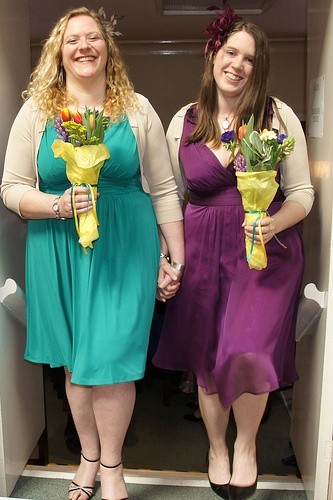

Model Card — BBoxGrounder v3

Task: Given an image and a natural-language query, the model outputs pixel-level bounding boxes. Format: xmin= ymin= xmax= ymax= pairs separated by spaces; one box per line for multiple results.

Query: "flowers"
xmin=51 ymin=104 xmax=112 ymax=255
xmin=219 ymin=112 xmax=296 ymax=269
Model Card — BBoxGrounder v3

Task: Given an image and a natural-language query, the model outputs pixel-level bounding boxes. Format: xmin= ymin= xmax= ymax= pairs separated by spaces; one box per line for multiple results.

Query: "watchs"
xmin=53 ymin=197 xmax=66 ymax=220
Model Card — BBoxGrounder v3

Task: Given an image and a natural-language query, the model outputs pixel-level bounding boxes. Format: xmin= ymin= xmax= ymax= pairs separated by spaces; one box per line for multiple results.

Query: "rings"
xmin=266 ymin=234 xmax=267 ymax=239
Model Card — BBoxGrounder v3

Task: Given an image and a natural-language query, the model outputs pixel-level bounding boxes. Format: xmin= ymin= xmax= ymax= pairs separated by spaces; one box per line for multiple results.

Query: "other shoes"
xmin=184 ymin=413 xmax=202 ymax=421
xmin=186 ymin=402 xmax=199 ymax=409
xmin=265 ymin=404 xmax=271 ymax=413
xmin=65 ymin=428 xmax=81 ymax=454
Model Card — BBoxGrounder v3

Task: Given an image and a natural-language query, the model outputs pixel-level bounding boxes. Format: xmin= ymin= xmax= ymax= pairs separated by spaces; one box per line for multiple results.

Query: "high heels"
xmin=99 ymin=462 xmax=128 ymax=500
xmin=206 ymin=448 xmax=231 ymax=500
xmin=228 ymin=447 xmax=258 ymax=500
xmin=68 ymin=454 xmax=100 ymax=500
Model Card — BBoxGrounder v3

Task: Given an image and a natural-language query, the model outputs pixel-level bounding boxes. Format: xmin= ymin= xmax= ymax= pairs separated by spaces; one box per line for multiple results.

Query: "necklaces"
xmin=218 ymin=115 xmax=233 ymax=129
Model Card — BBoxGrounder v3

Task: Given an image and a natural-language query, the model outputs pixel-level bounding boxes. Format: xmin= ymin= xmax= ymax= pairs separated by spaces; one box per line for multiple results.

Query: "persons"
xmin=156 ymin=5 xmax=314 ymax=500
xmin=0 ymin=6 xmax=185 ymax=500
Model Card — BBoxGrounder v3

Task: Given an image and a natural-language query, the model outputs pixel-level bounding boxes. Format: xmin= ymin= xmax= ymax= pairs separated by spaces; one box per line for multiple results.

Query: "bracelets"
xmin=171 ymin=261 xmax=185 ymax=271
xmin=160 ymin=252 xmax=169 ymax=261
